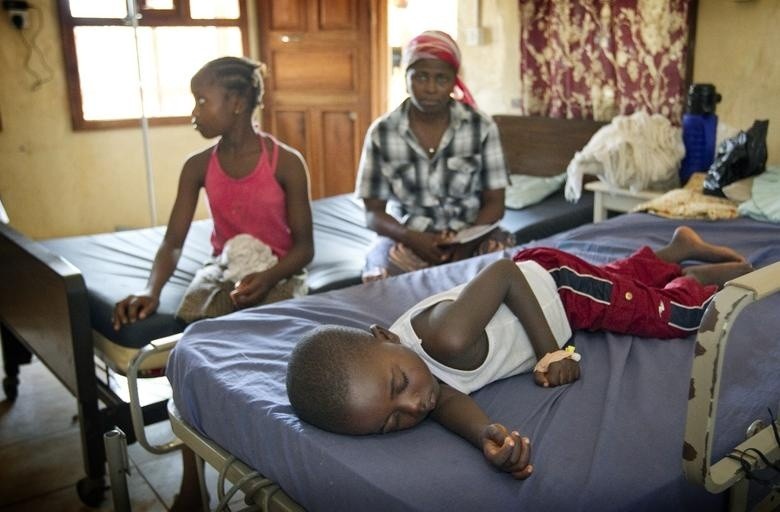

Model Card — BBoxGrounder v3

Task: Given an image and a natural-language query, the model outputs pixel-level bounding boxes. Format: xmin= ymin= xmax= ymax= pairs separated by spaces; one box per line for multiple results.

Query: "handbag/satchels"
xmin=581 ymin=123 xmax=680 ymax=190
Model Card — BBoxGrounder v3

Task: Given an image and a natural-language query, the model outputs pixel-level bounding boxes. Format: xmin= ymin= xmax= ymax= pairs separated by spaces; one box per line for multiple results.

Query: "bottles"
xmin=681 ymin=82 xmax=725 ymax=185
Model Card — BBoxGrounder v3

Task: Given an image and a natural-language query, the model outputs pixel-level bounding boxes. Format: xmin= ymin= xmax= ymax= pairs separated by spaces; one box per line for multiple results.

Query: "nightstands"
xmin=585 ymin=180 xmax=665 ymax=221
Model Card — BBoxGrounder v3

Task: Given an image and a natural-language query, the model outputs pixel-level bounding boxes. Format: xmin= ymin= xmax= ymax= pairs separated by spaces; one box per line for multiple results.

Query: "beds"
xmin=169 ymin=214 xmax=780 ymax=512
xmin=1 ymin=115 xmax=611 ymax=507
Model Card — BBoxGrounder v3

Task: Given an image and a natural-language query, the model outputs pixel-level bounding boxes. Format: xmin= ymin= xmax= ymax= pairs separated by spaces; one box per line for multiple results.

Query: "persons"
xmin=352 ymin=29 xmax=519 ymax=282
xmin=286 ymin=223 xmax=758 ymax=482
xmin=109 ymin=55 xmax=316 ymax=512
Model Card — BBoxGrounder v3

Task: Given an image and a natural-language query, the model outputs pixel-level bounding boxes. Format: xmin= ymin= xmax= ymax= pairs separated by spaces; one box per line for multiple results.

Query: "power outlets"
xmin=9 ymin=10 xmax=31 ymax=29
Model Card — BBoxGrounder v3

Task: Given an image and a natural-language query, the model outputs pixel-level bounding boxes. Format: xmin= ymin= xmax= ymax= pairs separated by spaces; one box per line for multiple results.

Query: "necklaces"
xmin=410 ymin=109 xmax=450 ymax=155
